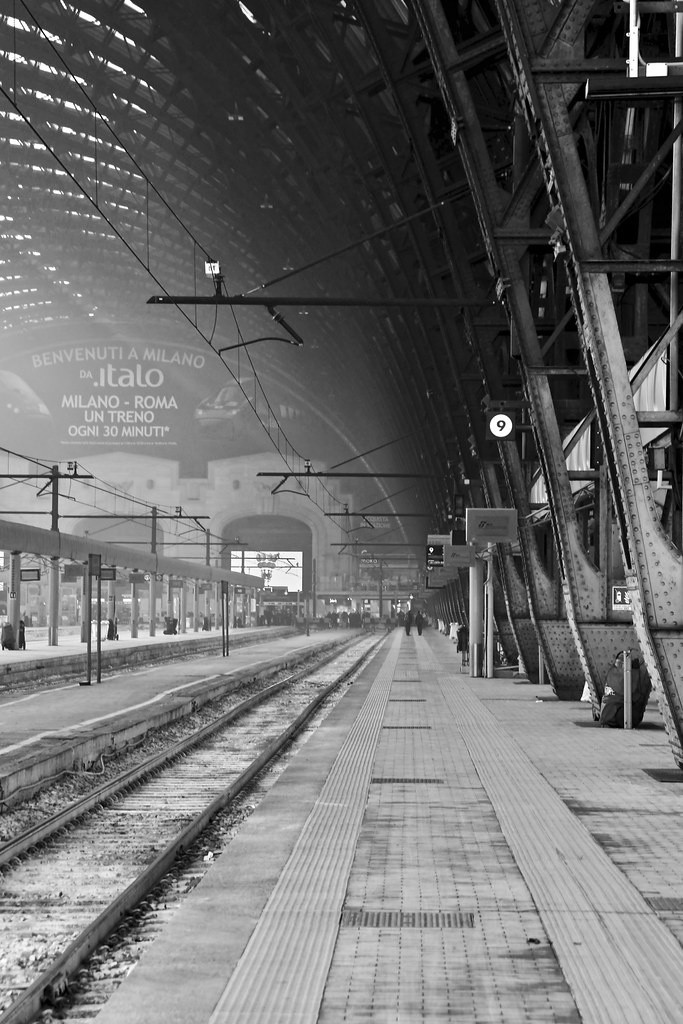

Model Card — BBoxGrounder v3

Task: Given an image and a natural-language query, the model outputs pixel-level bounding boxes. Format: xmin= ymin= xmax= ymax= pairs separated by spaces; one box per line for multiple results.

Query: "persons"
xmin=415 ymin=611 xmax=423 ymax=636
xmin=404 ymin=610 xmax=412 ymax=636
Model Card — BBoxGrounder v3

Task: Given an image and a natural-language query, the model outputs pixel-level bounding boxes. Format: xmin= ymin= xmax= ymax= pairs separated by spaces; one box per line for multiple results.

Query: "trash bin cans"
xmin=162 ymin=618 xmax=178 ymax=636
xmin=91 ymin=619 xmax=120 ymax=642
xmin=458 ymin=627 xmax=470 ymax=668
xmin=19 ymin=621 xmax=25 ymax=653
xmin=1 ymin=624 xmax=15 ymax=651
